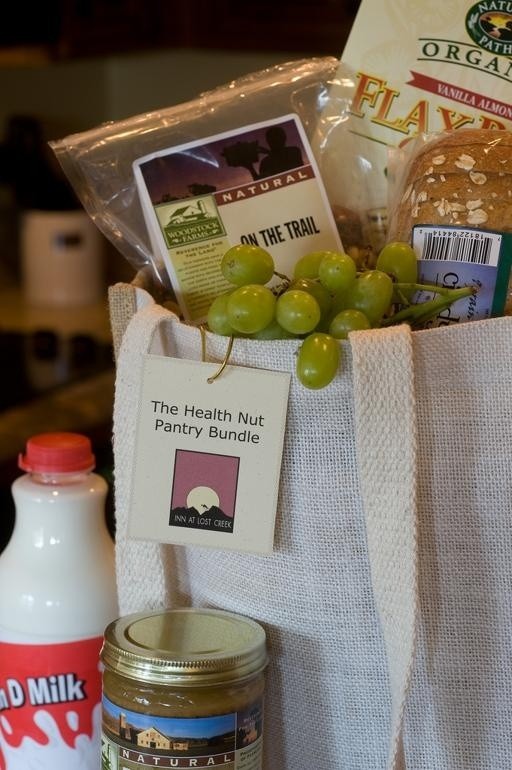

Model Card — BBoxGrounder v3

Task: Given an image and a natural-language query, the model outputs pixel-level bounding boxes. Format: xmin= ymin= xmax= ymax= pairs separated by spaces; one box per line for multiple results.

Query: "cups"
xmin=23 ymin=211 xmax=103 ymax=310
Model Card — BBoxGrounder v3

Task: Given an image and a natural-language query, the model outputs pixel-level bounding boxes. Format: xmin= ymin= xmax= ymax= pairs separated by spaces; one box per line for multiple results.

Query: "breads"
xmin=391 ymin=127 xmax=512 ymax=329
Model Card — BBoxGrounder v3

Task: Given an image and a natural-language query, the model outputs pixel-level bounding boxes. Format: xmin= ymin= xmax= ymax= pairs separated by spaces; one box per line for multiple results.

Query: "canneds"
xmin=100 ymin=608 xmax=268 ymax=770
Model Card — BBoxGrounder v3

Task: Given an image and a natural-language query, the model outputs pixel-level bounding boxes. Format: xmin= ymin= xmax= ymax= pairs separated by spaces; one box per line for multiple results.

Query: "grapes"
xmin=207 ymin=244 xmax=478 ymax=390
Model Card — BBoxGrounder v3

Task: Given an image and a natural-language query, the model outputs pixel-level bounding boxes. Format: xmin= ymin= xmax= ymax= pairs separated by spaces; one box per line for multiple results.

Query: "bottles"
xmin=0 ymin=432 xmax=117 ymax=770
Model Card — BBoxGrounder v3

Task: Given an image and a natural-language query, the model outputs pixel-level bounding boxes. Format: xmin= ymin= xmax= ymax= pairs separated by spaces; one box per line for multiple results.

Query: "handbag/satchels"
xmin=108 ymin=271 xmax=512 ymax=768
